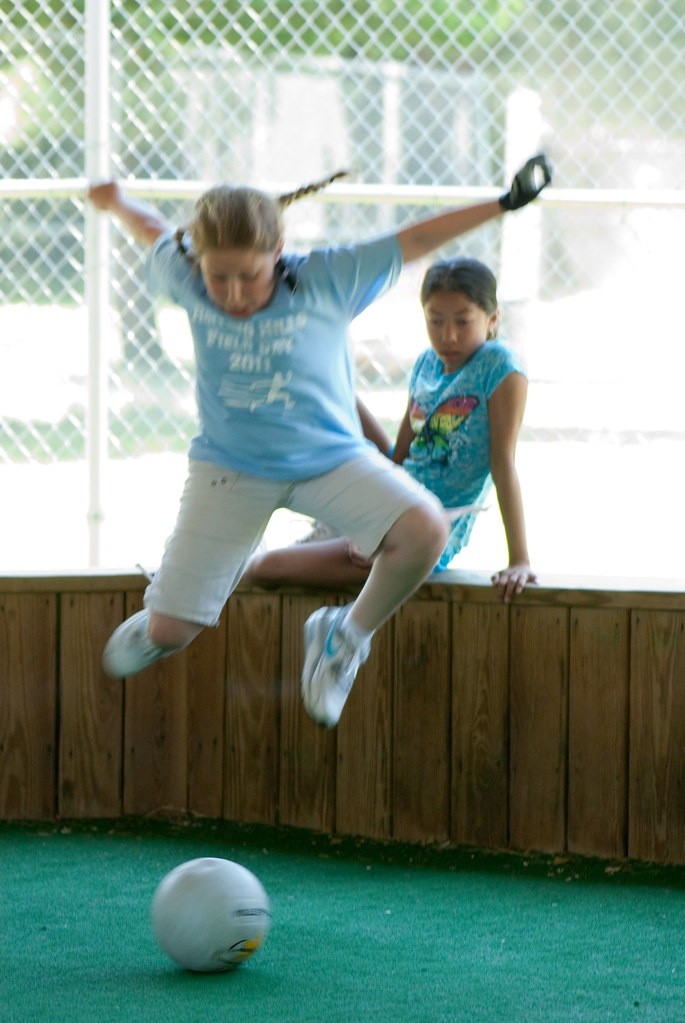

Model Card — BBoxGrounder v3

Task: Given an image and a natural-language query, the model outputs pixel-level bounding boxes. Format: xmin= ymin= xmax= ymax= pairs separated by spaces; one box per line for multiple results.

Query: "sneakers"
xmin=301 ymin=601 xmax=372 ymax=729
xmin=101 ymin=608 xmax=181 ymax=681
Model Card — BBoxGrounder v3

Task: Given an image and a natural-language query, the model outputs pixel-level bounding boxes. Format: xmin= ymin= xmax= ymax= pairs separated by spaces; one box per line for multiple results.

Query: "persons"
xmin=240 ymin=256 xmax=539 ymax=604
xmin=88 ymin=154 xmax=553 ymax=728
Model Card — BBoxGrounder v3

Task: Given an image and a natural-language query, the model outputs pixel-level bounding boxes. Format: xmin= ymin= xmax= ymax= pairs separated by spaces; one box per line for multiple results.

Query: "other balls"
xmin=152 ymin=857 xmax=271 ymax=973
